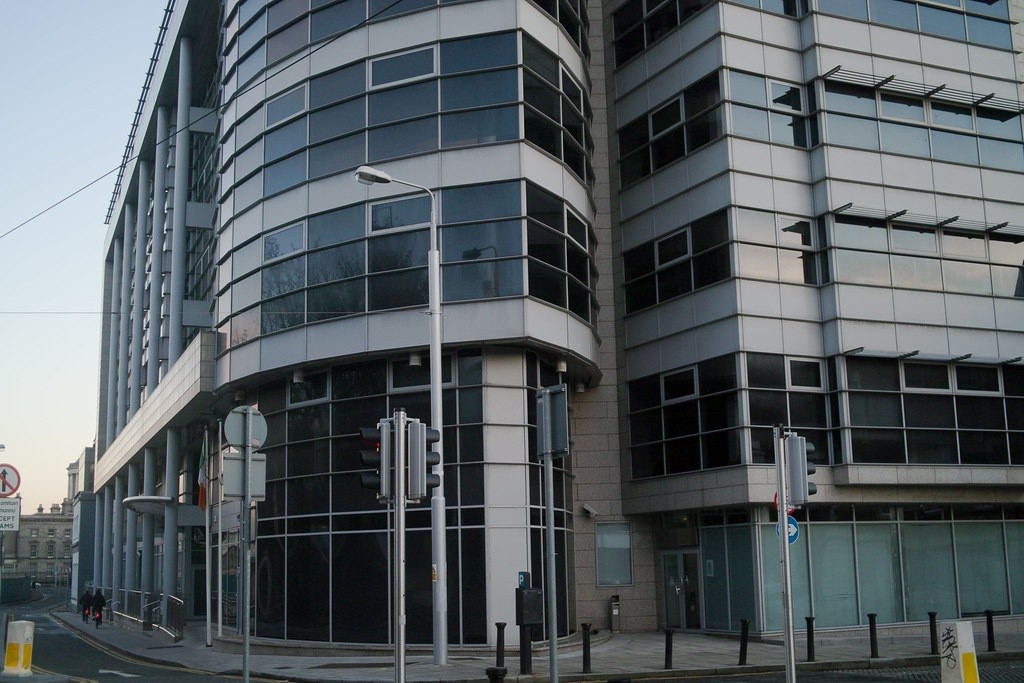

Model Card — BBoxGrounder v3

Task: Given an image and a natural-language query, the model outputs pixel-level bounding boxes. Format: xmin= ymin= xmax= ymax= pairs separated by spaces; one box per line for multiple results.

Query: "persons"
xmin=90 ymin=591 xmax=105 ymax=626
xmin=78 ymin=590 xmax=92 ymax=619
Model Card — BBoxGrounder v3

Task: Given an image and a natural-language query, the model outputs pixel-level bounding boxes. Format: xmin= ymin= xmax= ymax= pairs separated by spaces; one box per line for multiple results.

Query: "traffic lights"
xmin=357 ymin=418 xmax=393 ymax=505
xmin=406 ymin=420 xmax=443 ymax=500
xmin=787 ymin=436 xmax=819 ymax=508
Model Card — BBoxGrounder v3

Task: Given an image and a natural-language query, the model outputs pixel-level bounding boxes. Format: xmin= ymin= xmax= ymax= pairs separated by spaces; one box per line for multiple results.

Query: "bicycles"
xmin=94 ymin=610 xmax=102 ymax=629
xmin=82 ymin=605 xmax=90 ymax=624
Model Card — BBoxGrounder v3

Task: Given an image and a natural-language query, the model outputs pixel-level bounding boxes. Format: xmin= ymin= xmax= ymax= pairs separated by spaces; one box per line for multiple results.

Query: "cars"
xmin=29 ymin=580 xmax=42 ymax=589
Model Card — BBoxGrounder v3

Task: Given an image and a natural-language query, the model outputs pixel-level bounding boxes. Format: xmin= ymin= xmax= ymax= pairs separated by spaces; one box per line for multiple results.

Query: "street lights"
xmin=355 ymin=165 xmax=449 ymax=666
xmin=462 ymin=244 xmax=500 ymax=298
